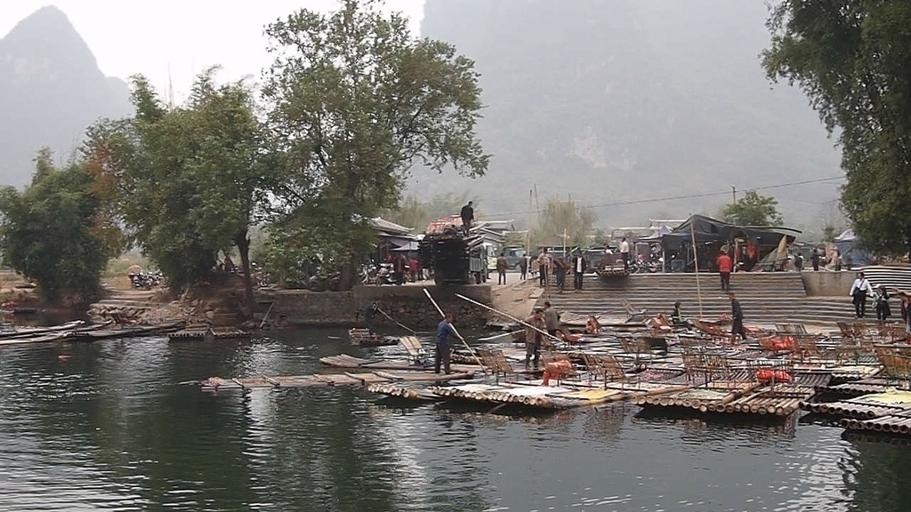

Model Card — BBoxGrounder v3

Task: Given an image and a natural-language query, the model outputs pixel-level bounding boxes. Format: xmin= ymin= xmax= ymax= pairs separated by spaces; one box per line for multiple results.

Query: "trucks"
xmin=434 ymin=245 xmax=533 ymax=286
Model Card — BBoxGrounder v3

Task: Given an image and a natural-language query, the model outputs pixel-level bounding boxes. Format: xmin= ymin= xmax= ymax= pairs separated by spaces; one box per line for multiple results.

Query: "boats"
xmin=596 ymin=262 xmax=630 ymax=278
xmin=0 ymin=303 xmax=212 ymax=344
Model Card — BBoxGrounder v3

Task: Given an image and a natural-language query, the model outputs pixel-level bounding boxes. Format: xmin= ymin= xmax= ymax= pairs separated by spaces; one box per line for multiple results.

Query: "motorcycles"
xmin=626 ymin=253 xmax=660 ymax=273
xmin=223 ymin=257 xmax=434 ymax=289
xmin=127 ymin=268 xmax=165 ymax=288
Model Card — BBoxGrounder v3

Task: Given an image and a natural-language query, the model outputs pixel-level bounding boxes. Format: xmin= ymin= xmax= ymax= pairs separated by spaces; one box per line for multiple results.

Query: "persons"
xmin=497 ymin=238 xmax=634 ymax=369
xmin=850 ymin=273 xmax=875 ymax=318
xmin=384 ymin=251 xmax=424 ymax=285
xmin=434 ymin=313 xmax=464 ymax=375
xmin=225 ymin=252 xmax=231 ymax=271
xmin=716 ymin=250 xmax=732 ymax=290
xmin=673 ymin=302 xmax=691 ymax=329
xmin=729 ymin=293 xmax=746 ymax=343
xmin=872 ymin=287 xmax=890 ymax=321
xmin=899 ymin=292 xmax=910 ymax=333
xmin=462 ymin=201 xmax=475 ymax=236
xmin=795 ymin=248 xmax=880 ymax=271
